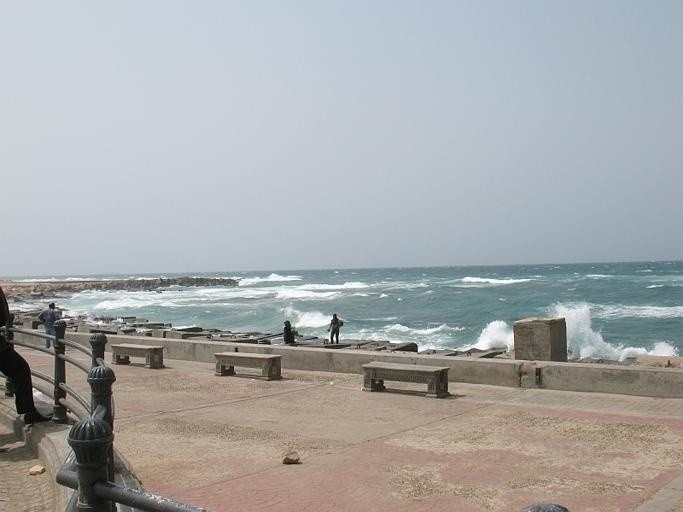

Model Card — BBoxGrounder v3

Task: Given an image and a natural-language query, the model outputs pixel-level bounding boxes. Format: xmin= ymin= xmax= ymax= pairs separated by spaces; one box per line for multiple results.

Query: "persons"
xmin=0 ymin=283 xmax=53 ymax=424
xmin=328 ymin=314 xmax=345 ymax=344
xmin=284 ymin=321 xmax=298 ymax=343
xmin=38 ymin=303 xmax=58 ymax=348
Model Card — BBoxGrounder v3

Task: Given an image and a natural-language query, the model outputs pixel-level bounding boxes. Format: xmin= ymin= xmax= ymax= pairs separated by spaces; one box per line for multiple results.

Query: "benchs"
xmin=360 ymin=361 xmax=451 ymax=399
xmin=213 ymin=351 xmax=285 ymax=382
xmin=110 ymin=343 xmax=165 ymax=369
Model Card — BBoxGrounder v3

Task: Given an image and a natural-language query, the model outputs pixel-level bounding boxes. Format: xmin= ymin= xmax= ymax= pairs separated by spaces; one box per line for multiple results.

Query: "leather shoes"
xmin=17 ymin=407 xmax=52 ymax=424
